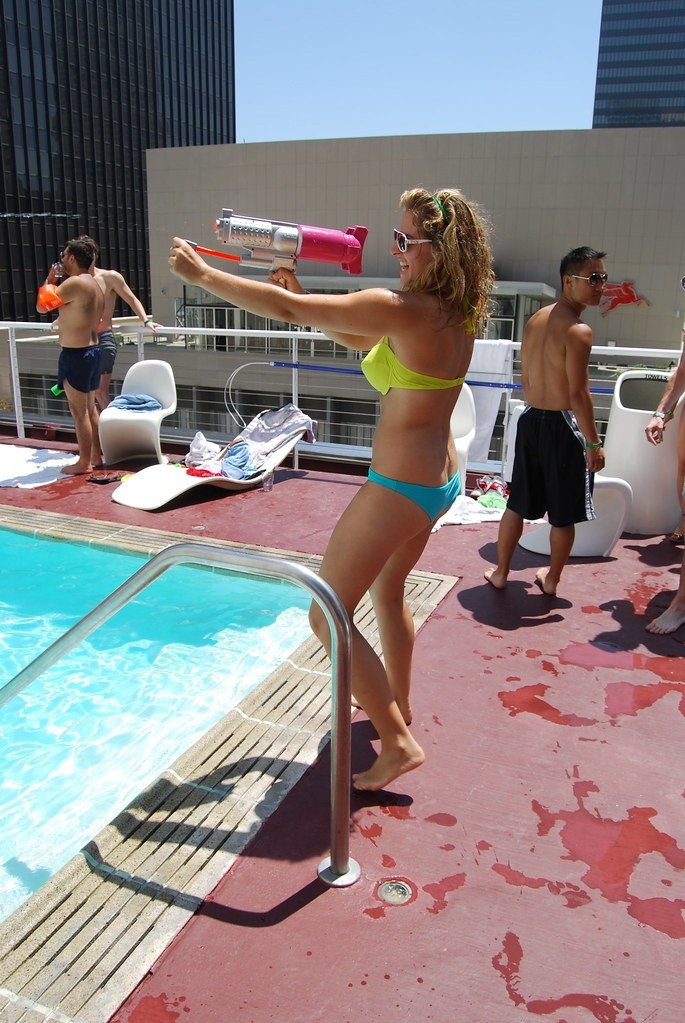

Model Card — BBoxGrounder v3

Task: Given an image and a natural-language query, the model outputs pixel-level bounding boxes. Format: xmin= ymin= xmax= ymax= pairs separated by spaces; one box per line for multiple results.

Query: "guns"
xmin=179 ymin=206 xmax=369 ymax=285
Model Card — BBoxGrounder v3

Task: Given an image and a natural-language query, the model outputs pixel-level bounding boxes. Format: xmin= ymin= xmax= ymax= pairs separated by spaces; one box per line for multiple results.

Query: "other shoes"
xmin=669 ymin=529 xmax=685 ymax=541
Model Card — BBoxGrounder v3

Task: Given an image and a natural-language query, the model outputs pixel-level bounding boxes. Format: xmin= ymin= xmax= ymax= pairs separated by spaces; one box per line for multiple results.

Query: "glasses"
xmin=393 ymin=229 xmax=433 ymax=252
xmin=60 ymin=252 xmax=70 ymax=258
xmin=572 ymin=273 xmax=608 ymax=287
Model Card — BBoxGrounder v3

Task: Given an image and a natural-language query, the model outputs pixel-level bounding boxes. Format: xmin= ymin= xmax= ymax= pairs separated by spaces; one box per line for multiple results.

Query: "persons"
xmin=168 ymin=190 xmax=494 ymax=791
xmin=51 ymin=236 xmax=164 ymax=412
xmin=484 ymin=246 xmax=608 ymax=594
xmin=644 ymin=274 xmax=685 ymax=635
xmin=36 ymin=235 xmax=106 ymax=474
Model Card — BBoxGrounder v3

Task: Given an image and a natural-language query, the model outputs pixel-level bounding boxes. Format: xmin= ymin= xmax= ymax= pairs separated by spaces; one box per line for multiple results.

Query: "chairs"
xmin=449 ymin=382 xmax=477 ymax=494
xmin=99 ymin=359 xmax=177 ymax=465
xmin=498 ymin=407 xmax=633 ymax=560
xmin=112 ymin=409 xmax=316 ymax=511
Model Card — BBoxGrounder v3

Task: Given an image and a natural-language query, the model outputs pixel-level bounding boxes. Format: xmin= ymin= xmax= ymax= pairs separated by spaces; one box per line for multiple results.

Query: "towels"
xmin=220 ymin=442 xmax=251 ymax=480
xmin=106 ymin=394 xmax=161 ymax=414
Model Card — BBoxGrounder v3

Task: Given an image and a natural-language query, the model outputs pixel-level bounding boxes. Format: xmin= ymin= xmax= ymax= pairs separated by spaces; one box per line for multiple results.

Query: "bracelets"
xmin=653 ymin=412 xmax=666 ymax=419
xmin=586 ymin=440 xmax=603 ymax=449
xmin=306 ymin=289 xmax=310 ymax=294
xmin=666 ymin=410 xmax=673 ymax=416
xmin=144 ymin=320 xmax=150 ymax=327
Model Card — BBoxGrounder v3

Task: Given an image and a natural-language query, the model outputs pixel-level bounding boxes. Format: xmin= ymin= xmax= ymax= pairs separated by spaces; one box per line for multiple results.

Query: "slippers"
xmin=85 ymin=472 xmax=122 ymax=484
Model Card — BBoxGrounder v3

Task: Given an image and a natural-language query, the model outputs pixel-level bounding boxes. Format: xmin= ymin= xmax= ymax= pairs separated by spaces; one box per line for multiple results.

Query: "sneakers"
xmin=470 ymin=475 xmax=508 ymax=498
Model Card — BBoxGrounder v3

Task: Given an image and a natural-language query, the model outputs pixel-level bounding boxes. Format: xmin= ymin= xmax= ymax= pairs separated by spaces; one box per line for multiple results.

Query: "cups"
xmin=53 ymin=262 xmax=65 ymax=278
xmin=262 ymin=472 xmax=275 ymax=493
